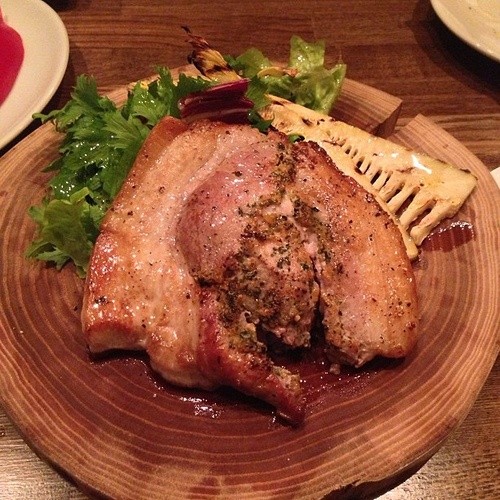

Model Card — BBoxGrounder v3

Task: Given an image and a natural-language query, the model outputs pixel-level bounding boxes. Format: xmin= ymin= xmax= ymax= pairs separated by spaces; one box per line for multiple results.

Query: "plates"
xmin=430 ymin=0 xmax=500 ymax=61
xmin=0 ymin=0 xmax=70 ymax=150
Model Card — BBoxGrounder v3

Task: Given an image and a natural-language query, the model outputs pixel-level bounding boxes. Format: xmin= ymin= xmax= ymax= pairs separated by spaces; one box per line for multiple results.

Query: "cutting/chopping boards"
xmin=0 ymin=57 xmax=500 ymax=500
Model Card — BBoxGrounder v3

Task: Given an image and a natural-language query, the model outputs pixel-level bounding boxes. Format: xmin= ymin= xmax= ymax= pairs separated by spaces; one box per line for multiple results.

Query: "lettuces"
xmin=25 ymin=35 xmax=348 ymax=279
